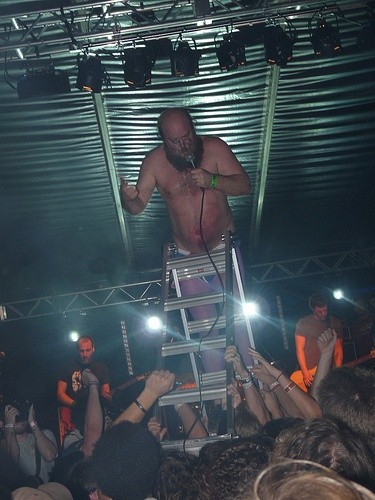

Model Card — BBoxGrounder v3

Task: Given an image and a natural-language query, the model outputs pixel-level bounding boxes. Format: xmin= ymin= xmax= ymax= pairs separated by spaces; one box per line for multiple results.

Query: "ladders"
xmin=153 ymin=231 xmax=262 ymax=457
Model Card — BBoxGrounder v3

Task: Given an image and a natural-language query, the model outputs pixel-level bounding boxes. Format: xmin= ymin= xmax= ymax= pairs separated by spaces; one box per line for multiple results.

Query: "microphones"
xmin=186 ymin=152 xmax=205 ymax=191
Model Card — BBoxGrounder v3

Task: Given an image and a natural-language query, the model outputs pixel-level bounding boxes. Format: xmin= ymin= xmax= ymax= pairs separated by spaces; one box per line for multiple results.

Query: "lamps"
xmin=0 ymin=0 xmax=375 ymax=96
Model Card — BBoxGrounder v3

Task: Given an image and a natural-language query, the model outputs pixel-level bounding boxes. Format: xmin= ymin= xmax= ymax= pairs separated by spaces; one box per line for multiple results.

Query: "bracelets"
xmin=276 ymin=370 xmax=285 ymax=381
xmin=272 ymin=384 xmax=282 ymax=392
xmin=173 ymin=403 xmax=183 ymax=412
xmin=132 ymin=398 xmax=147 ymax=415
xmin=210 ymin=174 xmax=217 ymax=188
xmin=284 ymin=381 xmax=297 ymax=393
xmin=270 ymin=381 xmax=279 ymax=389
xmin=88 ymin=380 xmax=99 ymax=386
xmin=241 ymin=381 xmax=253 ymax=389
xmin=29 ymin=420 xmax=38 ymax=427
xmin=4 ymin=423 xmax=15 ymax=430
xmin=235 ymin=373 xmax=252 ymax=385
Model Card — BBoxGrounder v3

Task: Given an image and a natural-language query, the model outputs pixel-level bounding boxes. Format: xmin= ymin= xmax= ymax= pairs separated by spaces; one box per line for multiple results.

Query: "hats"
xmin=7 ymin=399 xmax=31 ymax=423
xmin=11 ymin=481 xmax=74 ymax=500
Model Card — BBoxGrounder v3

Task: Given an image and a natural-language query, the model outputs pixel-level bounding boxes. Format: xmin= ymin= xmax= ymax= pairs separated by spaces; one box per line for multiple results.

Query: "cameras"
xmin=8 ymin=400 xmax=31 ymax=422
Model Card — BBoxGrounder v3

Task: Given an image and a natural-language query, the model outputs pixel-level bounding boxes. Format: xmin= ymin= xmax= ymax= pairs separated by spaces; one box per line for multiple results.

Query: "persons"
xmin=57 ymin=336 xmax=112 ymax=444
xmin=0 ymin=327 xmax=375 ymax=500
xmin=118 ymin=107 xmax=255 ymax=373
xmin=295 ymin=292 xmax=344 ymax=388
